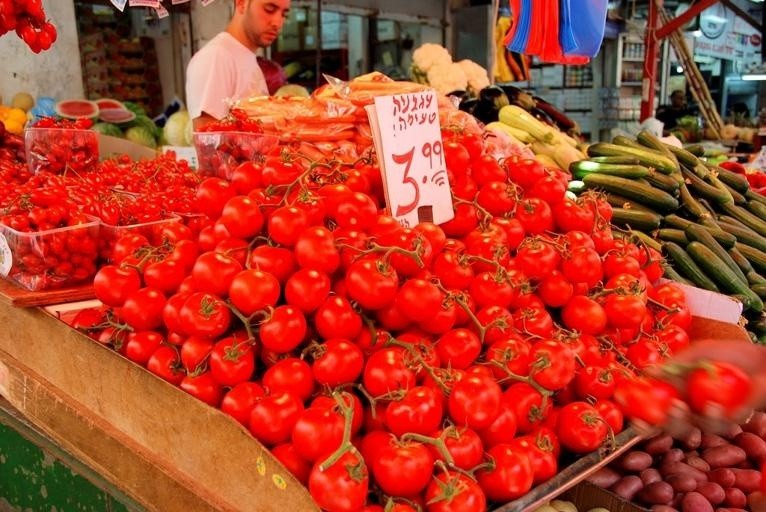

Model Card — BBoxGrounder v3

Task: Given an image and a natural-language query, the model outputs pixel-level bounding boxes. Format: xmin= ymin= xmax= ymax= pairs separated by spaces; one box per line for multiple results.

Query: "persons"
xmin=729 ymin=102 xmax=751 ymax=119
xmin=656 ymin=90 xmax=699 ymax=130
xmin=185 ymin=0 xmax=291 ymax=133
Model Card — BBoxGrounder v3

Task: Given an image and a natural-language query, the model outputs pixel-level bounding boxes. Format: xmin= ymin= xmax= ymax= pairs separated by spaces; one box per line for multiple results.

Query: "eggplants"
xmin=446 ymin=84 xmax=576 ymax=129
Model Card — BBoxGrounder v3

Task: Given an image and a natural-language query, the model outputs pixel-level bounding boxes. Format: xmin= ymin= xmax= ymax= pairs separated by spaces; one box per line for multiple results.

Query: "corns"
xmin=487 ymin=105 xmax=592 ymax=173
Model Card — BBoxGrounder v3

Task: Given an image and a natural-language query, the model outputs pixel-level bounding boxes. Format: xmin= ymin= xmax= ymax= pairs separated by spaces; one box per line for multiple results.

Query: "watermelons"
xmin=55 ymin=97 xmax=160 ymax=152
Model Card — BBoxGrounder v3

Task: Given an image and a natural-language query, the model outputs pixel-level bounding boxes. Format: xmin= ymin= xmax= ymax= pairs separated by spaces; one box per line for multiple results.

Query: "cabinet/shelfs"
xmin=611 ymin=33 xmax=669 ymax=105
xmin=0 ymin=275 xmax=766 ymax=512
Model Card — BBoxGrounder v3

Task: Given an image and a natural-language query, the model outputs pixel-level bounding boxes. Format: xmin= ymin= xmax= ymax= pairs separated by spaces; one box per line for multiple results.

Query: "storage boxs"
xmin=113 ymin=178 xmax=178 ymax=202
xmin=176 ymin=206 xmax=209 ymax=242
xmin=99 ymin=217 xmax=178 ymax=267
xmin=0 ymin=205 xmax=101 ymax=290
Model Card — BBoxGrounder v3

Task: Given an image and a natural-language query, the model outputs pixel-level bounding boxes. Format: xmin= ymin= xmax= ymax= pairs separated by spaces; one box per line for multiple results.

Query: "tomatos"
xmin=0 ymin=117 xmax=757 ymax=511
xmin=0 ymin=0 xmax=56 ymax=54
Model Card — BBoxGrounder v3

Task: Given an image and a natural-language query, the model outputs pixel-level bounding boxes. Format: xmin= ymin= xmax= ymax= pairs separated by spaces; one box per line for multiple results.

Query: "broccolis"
xmin=411 ymin=43 xmax=491 ymax=96
